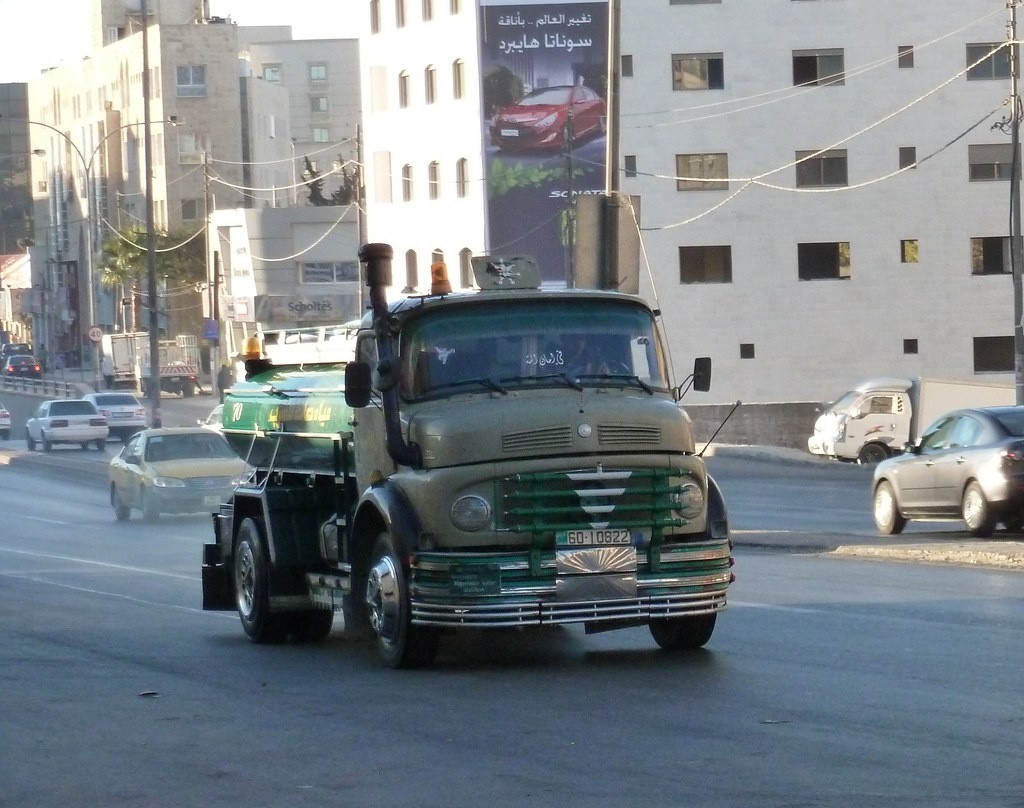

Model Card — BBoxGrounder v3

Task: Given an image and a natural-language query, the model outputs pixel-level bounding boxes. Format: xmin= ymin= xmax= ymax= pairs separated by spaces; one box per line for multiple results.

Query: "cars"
xmin=0 ymin=401 xmax=11 ymax=440
xmin=82 ymin=393 xmax=147 ymax=441
xmin=107 ymin=427 xmax=255 ymax=525
xmin=197 ymin=402 xmax=226 ymax=442
xmin=491 ymin=85 xmax=607 ymax=153
xmin=25 ymin=400 xmax=109 ymax=452
xmin=0 ymin=343 xmax=34 ymax=368
xmin=2 ymin=355 xmax=41 ymax=379
xmin=872 ymin=406 xmax=1024 ymax=537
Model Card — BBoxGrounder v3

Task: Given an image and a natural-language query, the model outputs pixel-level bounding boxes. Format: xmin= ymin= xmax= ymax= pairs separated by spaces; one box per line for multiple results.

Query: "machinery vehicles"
xmin=199 ymin=239 xmax=735 ymax=668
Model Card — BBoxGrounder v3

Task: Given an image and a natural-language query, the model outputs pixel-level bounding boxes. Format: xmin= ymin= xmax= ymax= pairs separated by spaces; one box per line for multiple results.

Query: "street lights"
xmin=1 ymin=114 xmax=186 ymax=392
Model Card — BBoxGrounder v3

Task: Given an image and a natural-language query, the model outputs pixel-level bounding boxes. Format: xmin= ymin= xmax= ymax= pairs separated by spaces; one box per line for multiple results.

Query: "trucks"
xmin=807 ymin=373 xmax=1015 ymax=464
xmin=133 ymin=333 xmax=199 ymax=397
xmin=99 ymin=333 xmax=149 ymax=385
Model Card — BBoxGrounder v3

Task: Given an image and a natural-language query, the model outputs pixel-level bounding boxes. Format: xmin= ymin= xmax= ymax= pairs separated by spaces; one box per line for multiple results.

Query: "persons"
xmin=218 ymin=364 xmax=231 ymax=403
xmin=549 ymin=330 xmax=610 ymax=375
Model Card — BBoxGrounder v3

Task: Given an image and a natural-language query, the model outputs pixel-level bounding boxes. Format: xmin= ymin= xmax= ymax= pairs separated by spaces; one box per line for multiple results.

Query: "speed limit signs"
xmin=88 ymin=325 xmax=103 ymax=342
